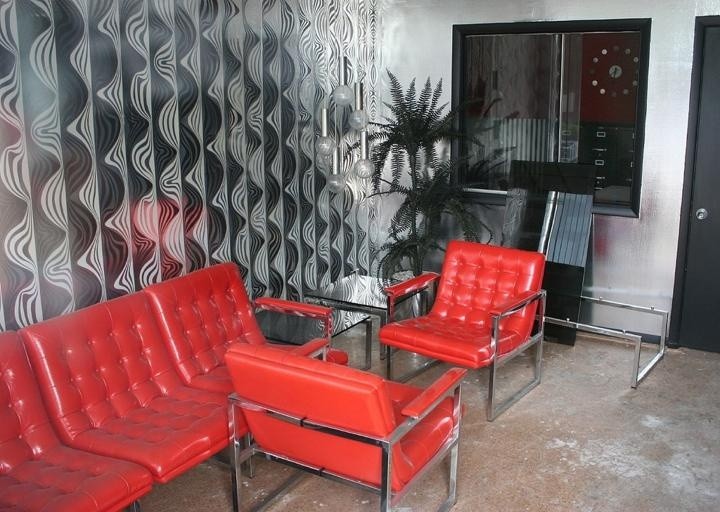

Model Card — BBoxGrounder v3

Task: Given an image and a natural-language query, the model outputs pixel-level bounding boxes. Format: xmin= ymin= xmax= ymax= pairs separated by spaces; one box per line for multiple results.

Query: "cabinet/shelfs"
xmin=580 ymin=118 xmax=633 ymax=193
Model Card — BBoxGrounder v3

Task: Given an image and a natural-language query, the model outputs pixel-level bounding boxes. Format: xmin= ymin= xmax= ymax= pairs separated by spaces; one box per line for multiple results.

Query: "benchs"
xmin=0 ymin=262 xmax=329 ymax=512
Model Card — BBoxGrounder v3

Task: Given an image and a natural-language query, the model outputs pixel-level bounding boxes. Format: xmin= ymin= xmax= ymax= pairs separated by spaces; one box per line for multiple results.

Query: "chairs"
xmin=205 ymin=336 xmax=470 ymax=511
xmin=380 ymin=239 xmax=549 ymax=424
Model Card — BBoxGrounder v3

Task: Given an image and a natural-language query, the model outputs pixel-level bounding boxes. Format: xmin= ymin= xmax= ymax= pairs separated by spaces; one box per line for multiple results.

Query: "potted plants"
xmin=350 ymin=65 xmax=522 ymax=348
